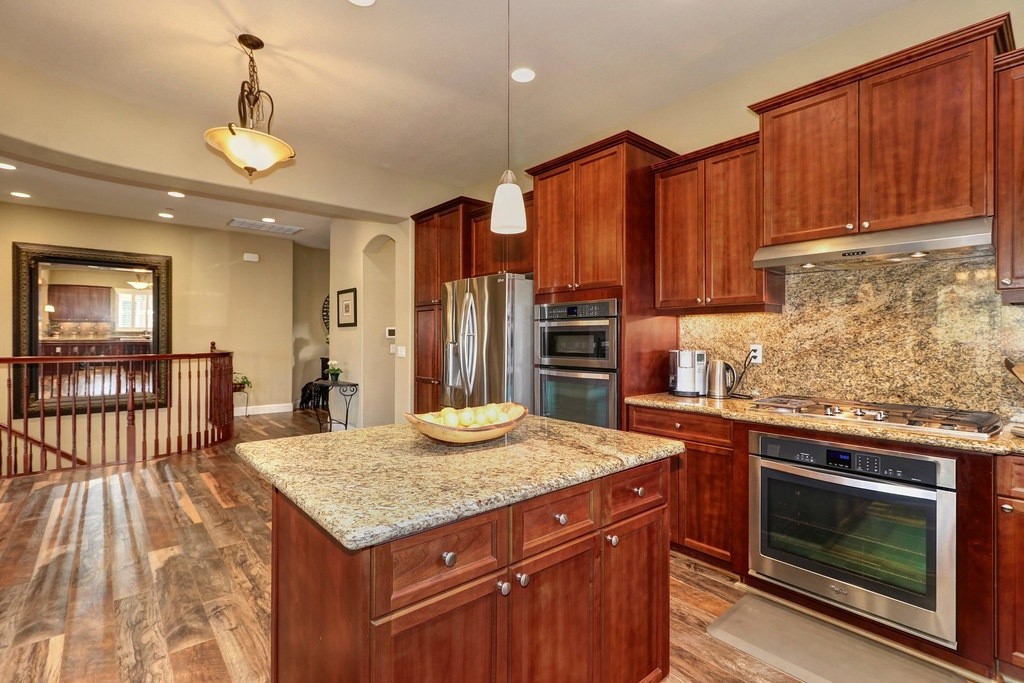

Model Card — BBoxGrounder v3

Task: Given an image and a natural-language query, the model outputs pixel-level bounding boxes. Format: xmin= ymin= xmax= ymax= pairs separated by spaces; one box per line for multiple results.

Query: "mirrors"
xmin=12 ymin=241 xmax=172 ymax=419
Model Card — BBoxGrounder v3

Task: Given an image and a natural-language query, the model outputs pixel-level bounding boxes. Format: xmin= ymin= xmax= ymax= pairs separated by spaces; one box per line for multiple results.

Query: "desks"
xmin=311 ymin=380 xmax=359 ymax=433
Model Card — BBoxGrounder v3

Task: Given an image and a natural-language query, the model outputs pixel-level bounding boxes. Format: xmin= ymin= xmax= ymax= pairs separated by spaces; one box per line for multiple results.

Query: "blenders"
xmin=668 ymin=349 xmax=707 ymax=397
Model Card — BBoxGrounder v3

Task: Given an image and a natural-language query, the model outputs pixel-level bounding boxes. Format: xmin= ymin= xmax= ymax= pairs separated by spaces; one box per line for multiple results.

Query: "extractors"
xmin=753 ymin=216 xmax=996 ymax=276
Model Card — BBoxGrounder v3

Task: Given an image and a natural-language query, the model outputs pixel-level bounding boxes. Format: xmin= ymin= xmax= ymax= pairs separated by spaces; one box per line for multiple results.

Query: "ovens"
xmin=532 ymin=298 xmax=618 ymax=430
xmin=748 ymin=429 xmax=957 ymax=651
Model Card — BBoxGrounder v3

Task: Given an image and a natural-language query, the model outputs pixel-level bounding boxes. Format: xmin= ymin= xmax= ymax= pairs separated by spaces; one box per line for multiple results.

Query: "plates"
xmin=402 ymin=402 xmax=528 ymax=443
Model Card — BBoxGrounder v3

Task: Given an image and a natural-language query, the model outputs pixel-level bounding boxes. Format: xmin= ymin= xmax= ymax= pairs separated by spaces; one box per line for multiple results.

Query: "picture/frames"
xmin=337 ymin=287 xmax=357 ymax=327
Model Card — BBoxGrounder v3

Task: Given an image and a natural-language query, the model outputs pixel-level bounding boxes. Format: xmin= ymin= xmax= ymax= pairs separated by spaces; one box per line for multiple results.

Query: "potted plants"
xmin=232 ymin=371 xmax=252 ymax=392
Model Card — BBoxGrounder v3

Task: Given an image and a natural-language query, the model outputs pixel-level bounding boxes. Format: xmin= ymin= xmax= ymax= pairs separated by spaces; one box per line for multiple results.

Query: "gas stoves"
xmin=748 ymin=394 xmax=1003 ymax=438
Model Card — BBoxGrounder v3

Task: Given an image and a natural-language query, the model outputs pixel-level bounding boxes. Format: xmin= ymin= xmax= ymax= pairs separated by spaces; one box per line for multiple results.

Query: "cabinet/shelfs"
xmin=41 ymin=344 xmax=151 ymax=376
xmin=534 ymin=143 xmax=667 ymax=294
xmin=995 ymin=454 xmax=1024 ymax=681
xmin=472 ymin=189 xmax=534 ymax=279
xmin=269 ymin=459 xmax=671 ymax=683
xmin=91 ymin=287 xmax=118 ymax=322
xmin=414 ymin=379 xmax=442 ymax=414
xmin=414 ymin=304 xmax=444 ymax=381
xmin=70 ymin=286 xmax=90 ymax=322
xmin=758 ymin=36 xmax=1003 ymax=248
xmin=654 ymin=142 xmax=786 ymax=317
xmin=996 ymin=64 xmax=1024 ymax=293
xmin=413 ymin=204 xmax=475 ymax=306
xmin=628 ymin=405 xmax=735 ymax=563
xmin=48 ymin=286 xmax=70 ymax=322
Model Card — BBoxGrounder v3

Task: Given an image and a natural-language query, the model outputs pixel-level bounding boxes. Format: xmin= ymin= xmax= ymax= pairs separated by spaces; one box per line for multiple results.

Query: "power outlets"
xmin=749 ymin=345 xmax=762 ymax=364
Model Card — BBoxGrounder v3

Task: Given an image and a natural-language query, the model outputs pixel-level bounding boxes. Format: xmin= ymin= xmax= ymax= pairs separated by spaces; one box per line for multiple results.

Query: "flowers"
xmin=325 ymin=360 xmax=342 ymax=373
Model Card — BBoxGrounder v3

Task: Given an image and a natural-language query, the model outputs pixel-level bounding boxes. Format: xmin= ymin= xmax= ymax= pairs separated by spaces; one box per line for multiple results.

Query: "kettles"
xmin=705 ymin=359 xmax=738 ymax=399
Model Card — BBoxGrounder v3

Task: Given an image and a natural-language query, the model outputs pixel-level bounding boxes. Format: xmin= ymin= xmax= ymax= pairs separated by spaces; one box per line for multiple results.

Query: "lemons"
xmin=421 ymin=403 xmax=510 ymax=427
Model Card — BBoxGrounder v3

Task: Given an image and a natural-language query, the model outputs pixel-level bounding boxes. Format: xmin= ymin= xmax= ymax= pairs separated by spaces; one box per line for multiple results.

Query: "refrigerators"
xmin=439 ymin=272 xmax=535 ymax=421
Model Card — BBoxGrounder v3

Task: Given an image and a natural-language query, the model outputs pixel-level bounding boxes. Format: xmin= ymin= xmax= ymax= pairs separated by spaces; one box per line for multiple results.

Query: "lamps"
xmin=489 ymin=0 xmax=528 ymax=234
xmin=44 ymin=270 xmax=55 ymax=313
xmin=204 ymin=33 xmax=297 ymax=177
xmin=126 ymin=273 xmax=153 ymax=290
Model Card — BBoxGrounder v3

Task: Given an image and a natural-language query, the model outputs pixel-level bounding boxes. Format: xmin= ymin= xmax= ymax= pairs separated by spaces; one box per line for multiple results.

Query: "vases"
xmin=330 ymin=373 xmax=339 ymax=382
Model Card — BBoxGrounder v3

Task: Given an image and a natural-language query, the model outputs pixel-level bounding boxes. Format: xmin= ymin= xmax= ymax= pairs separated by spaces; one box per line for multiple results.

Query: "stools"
xmin=234 ymin=390 xmax=251 ymax=418
